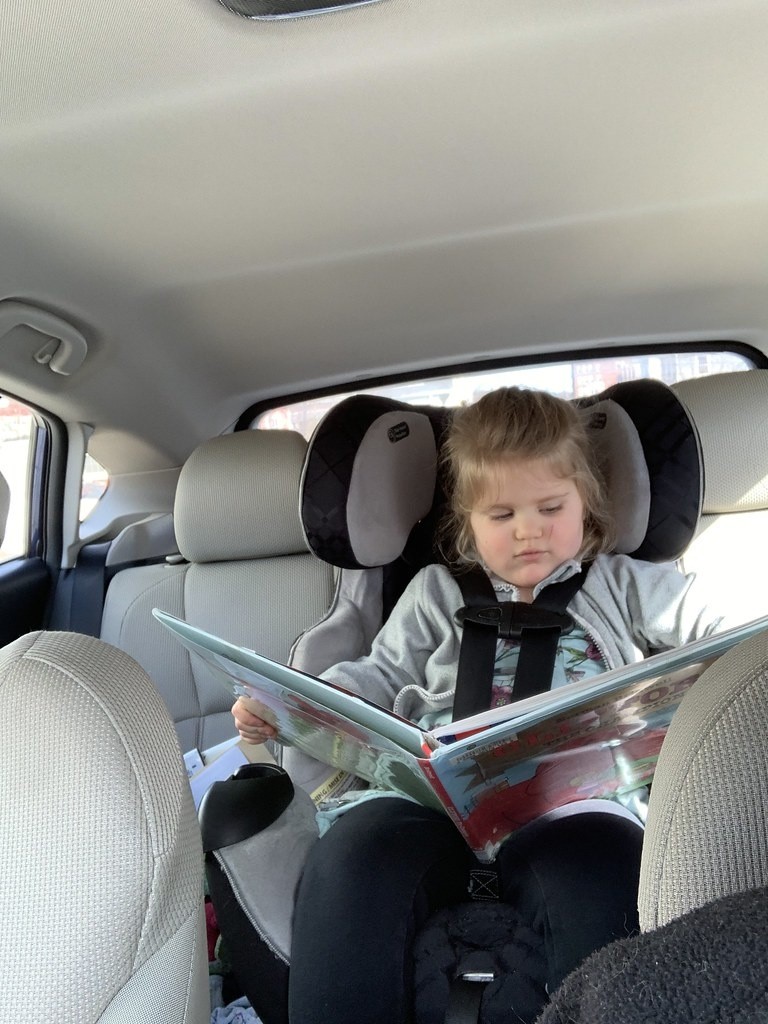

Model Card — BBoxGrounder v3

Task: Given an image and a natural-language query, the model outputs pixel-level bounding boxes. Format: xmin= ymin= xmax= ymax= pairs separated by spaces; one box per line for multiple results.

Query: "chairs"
xmin=0 ymin=372 xmax=768 ymax=1024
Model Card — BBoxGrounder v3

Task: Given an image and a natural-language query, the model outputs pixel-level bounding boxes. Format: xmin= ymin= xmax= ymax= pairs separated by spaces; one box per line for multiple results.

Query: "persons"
xmin=233 ymin=387 xmax=741 ymax=1024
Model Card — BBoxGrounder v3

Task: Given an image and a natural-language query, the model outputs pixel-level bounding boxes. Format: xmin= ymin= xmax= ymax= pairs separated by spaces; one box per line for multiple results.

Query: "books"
xmin=151 ymin=606 xmax=768 ymax=864
